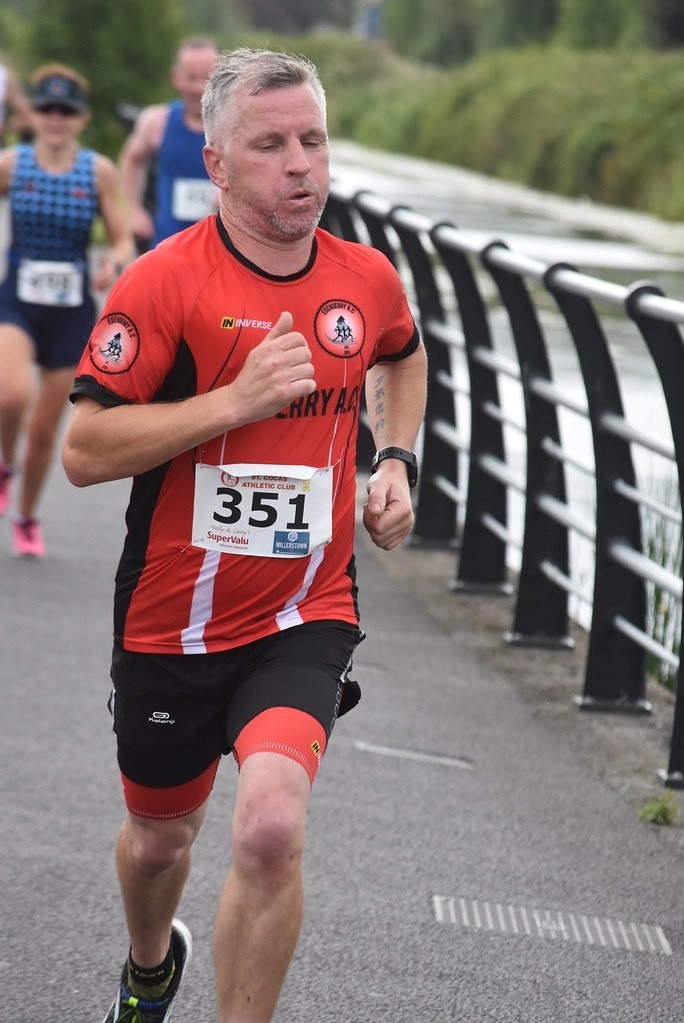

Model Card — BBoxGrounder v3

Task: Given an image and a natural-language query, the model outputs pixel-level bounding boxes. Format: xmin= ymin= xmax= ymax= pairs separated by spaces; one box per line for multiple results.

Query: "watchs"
xmin=370 ymin=446 xmax=419 ymax=488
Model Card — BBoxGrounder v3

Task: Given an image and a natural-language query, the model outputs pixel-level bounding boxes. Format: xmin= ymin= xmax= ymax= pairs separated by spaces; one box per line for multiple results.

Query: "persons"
xmin=117 ymin=37 xmax=222 ymax=256
xmin=0 ymin=63 xmax=138 ymax=561
xmin=0 ymin=62 xmax=36 ymax=149
xmin=61 ymin=47 xmax=425 ymax=1022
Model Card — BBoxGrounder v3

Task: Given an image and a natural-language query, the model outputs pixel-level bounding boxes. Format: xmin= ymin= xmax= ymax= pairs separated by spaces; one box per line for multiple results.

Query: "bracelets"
xmin=114 ymin=260 xmax=124 ymax=273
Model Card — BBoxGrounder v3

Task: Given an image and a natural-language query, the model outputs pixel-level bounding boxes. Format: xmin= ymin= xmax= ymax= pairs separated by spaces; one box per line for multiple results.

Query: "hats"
xmin=26 ymin=62 xmax=90 ymax=111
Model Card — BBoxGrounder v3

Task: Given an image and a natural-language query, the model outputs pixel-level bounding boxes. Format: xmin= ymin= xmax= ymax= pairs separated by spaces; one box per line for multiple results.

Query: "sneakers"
xmin=11 ymin=517 xmax=46 ymax=560
xmin=99 ymin=917 xmax=193 ymax=1023
xmin=0 ymin=463 xmax=16 ymax=516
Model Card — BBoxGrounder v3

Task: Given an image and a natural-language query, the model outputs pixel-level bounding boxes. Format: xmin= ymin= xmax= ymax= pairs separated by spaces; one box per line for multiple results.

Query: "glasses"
xmin=37 ymin=103 xmax=78 ymax=116
